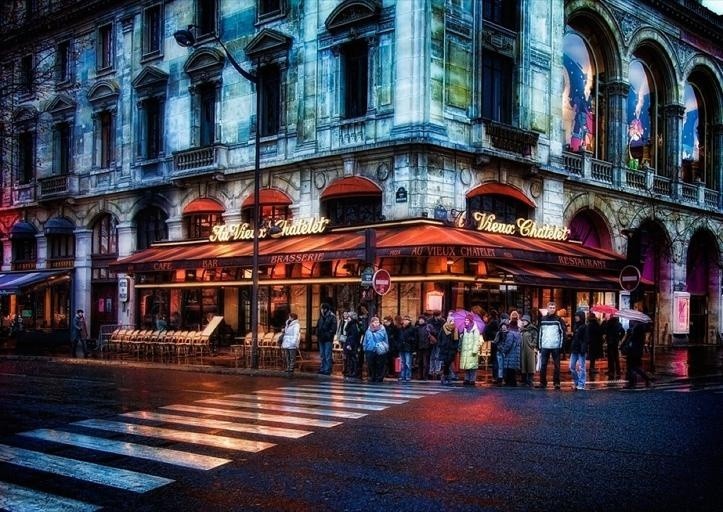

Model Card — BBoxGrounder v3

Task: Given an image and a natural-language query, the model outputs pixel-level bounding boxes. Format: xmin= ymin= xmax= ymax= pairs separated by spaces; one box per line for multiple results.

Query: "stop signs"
xmin=373 ymin=268 xmax=390 ymax=297
xmin=618 ymin=264 xmax=639 ymax=291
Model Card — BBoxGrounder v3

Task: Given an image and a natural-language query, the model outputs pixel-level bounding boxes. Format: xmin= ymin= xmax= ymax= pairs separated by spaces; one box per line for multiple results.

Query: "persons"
xmin=154 ymin=313 xmax=168 ymax=330
xmin=69 ymin=309 xmax=89 ymax=359
xmin=277 ymin=325 xmax=285 ymax=344
xmin=316 ymin=302 xmax=337 ymax=376
xmin=280 ymin=312 xmax=302 ymax=373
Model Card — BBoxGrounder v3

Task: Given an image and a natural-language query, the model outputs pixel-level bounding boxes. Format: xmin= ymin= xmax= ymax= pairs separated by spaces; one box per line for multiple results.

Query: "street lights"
xmin=174 ymin=24 xmax=261 ymax=370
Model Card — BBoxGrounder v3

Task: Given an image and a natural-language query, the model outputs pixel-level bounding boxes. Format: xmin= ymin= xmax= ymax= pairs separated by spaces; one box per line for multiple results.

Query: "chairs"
xmin=92 ymin=319 xmax=304 ymax=382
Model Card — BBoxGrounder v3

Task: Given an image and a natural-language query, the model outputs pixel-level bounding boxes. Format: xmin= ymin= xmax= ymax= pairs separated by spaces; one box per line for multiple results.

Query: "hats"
xmin=464 ymin=313 xmax=474 ymax=323
xmin=521 ymin=314 xmax=532 ymax=325
xmin=401 ymin=315 xmax=413 ymax=321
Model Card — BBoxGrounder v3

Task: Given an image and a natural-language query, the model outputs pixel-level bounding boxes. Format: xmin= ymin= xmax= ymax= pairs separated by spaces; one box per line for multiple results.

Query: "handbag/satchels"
xmin=375 ymin=341 xmax=391 ymax=357
xmin=278 ymin=332 xmax=284 ymax=345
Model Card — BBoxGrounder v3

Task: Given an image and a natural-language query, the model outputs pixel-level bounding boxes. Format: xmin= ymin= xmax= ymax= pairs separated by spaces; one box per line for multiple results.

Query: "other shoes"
xmin=286 ymin=368 xmax=295 ymax=372
xmin=318 ymin=371 xmax=428 ymax=384
xmin=643 ymin=383 xmax=655 ymax=391
xmin=624 ymin=383 xmax=636 ymax=390
xmin=441 ymin=374 xmax=533 ymax=387
xmin=553 ymin=382 xmax=561 ymax=388
xmin=535 ymin=382 xmax=547 ymax=388
xmin=573 ymin=383 xmax=585 ymax=391
